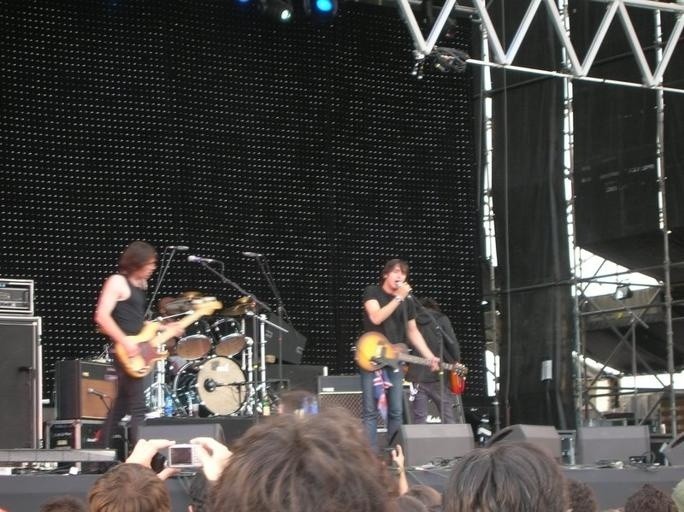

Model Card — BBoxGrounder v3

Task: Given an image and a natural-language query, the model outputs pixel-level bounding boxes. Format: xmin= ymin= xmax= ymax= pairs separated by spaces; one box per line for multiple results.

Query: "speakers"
xmin=388 ymin=423 xmax=475 ymax=466
xmin=136 ymin=423 xmax=225 ymax=459
xmin=259 ymin=364 xmax=328 ymax=394
xmin=55 ymin=360 xmax=121 ymax=420
xmin=0 ymin=316 xmax=42 ymax=449
xmin=313 ymin=375 xmax=362 ymax=393
xmin=575 ymin=424 xmax=650 ymax=465
xmin=485 ymin=425 xmax=561 ymax=458
xmin=246 ymin=313 xmax=307 ymax=364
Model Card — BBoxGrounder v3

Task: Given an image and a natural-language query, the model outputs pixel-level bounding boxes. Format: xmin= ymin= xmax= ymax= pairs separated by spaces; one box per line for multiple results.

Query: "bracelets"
xmin=395 ymin=295 xmax=402 ymax=303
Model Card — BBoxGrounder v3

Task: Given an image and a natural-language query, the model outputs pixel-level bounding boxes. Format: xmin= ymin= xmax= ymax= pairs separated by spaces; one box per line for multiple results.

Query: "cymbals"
xmin=222 ymin=295 xmax=255 ymax=315
xmin=164 ymin=292 xmax=223 ymax=309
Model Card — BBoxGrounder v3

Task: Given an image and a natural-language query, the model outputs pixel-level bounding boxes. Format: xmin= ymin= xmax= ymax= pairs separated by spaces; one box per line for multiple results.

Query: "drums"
xmin=208 ymin=317 xmax=246 ymax=357
xmin=174 ymin=316 xmax=214 ymax=360
xmin=173 ymin=356 xmax=249 ymax=417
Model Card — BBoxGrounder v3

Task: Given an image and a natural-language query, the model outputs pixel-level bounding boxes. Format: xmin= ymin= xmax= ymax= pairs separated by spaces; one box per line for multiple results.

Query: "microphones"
xmin=396 ymin=282 xmax=411 ymax=299
xmin=168 ymin=246 xmax=189 ymax=250
xmin=241 ymin=252 xmax=262 ymax=257
xmin=187 ymin=255 xmax=215 ymax=263
xmin=87 ymin=388 xmax=106 ymax=398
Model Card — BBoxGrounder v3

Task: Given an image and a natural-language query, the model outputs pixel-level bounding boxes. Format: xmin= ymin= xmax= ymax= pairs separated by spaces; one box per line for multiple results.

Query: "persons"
xmin=275 ymin=390 xmax=310 ymax=416
xmin=672 ymin=479 xmax=684 ymax=512
xmin=441 ymin=438 xmax=569 ymax=512
xmin=40 ymin=462 xmax=171 ymax=511
xmin=200 ymin=408 xmax=403 ymax=512
xmin=623 ymin=482 xmax=678 ymax=512
xmin=378 ymin=443 xmax=596 ymax=512
xmin=403 ymin=296 xmax=466 ymax=426
xmin=92 ymin=239 xmax=185 ymax=465
xmin=122 ymin=433 xmax=233 ymax=511
xmin=357 ymin=257 xmax=441 ymax=470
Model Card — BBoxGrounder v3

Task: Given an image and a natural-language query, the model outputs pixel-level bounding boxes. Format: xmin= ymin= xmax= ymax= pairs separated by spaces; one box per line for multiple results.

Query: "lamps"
xmin=272 ymin=4 xmax=292 ymax=23
xmin=612 ymin=284 xmax=634 ymax=300
xmin=314 ymin=0 xmax=337 ymax=16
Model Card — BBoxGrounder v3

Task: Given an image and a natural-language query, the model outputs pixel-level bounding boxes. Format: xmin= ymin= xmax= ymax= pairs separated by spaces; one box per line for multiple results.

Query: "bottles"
xmin=261 ymin=394 xmax=270 ymax=417
xmin=301 ymin=394 xmax=317 ymax=415
xmin=163 ymin=395 xmax=175 ymax=416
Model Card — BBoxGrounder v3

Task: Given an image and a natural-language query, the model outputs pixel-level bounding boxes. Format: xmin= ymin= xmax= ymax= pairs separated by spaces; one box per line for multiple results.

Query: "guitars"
xmin=450 ymin=371 xmax=464 ymax=394
xmin=116 ymin=300 xmax=223 ymax=378
xmin=355 ymin=331 xmax=468 ymax=377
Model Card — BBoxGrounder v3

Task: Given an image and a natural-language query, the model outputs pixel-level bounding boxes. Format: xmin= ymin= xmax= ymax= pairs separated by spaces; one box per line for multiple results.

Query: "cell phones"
xmin=168 ymin=444 xmax=194 ymax=468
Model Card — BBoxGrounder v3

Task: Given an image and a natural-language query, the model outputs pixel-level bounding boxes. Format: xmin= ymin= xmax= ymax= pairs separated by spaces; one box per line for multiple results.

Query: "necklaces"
xmin=133 ymin=277 xmax=149 ymax=294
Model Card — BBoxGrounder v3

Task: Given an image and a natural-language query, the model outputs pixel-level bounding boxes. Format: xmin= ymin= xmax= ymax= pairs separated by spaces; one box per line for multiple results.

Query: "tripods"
xmin=143 ymin=313 xmax=281 ymax=415
